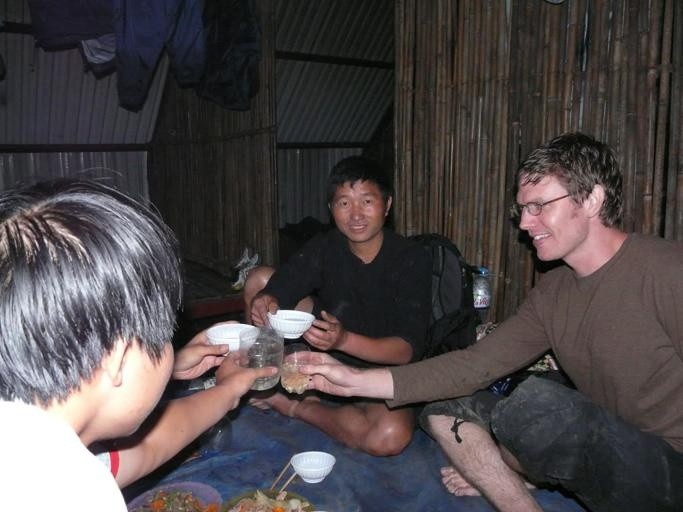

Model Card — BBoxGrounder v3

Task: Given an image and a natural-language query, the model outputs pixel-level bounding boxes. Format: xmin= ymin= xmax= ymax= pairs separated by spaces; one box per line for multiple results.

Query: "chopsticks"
xmin=270 ymin=455 xmax=300 ymax=495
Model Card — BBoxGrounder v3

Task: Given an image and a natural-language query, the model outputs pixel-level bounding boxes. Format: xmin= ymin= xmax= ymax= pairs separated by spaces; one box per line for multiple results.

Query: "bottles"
xmin=469 ymin=267 xmax=492 ymax=343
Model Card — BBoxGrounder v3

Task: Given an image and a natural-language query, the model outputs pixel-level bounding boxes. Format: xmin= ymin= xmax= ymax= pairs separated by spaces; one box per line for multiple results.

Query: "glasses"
xmin=512 ymin=190 xmax=579 ymax=216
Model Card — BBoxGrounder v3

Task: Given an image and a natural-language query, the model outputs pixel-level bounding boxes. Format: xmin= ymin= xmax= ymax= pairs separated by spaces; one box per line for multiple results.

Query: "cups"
xmin=238 ymin=326 xmax=284 ymax=390
xmin=280 ymin=343 xmax=310 ymax=396
xmin=200 ymin=410 xmax=233 ymax=452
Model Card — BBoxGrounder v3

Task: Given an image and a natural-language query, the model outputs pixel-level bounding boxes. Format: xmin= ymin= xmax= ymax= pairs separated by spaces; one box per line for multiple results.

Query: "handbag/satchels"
xmin=407 ymin=234 xmax=475 ymax=358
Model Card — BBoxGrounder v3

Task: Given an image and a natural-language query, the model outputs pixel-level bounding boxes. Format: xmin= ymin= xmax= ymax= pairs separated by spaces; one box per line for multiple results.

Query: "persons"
xmin=172 ymin=318 xmax=239 ymax=382
xmin=240 ymin=152 xmax=430 ymax=457
xmin=0 ymin=171 xmax=279 ymax=512
xmin=285 ymin=132 xmax=681 ymax=512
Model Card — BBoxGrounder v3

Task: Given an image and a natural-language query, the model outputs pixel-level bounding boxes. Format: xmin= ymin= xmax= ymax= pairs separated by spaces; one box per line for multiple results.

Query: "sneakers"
xmin=230 ymin=248 xmax=262 ymax=290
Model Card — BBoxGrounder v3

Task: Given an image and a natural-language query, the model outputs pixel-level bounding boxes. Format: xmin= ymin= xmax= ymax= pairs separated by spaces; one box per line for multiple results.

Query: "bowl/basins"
xmin=203 ymin=322 xmax=260 ymax=358
xmin=291 ymin=450 xmax=337 ymax=485
xmin=125 ymin=479 xmax=222 ymax=511
xmin=222 ymin=488 xmax=314 ymax=511
xmin=265 ymin=309 xmax=315 ymax=340
